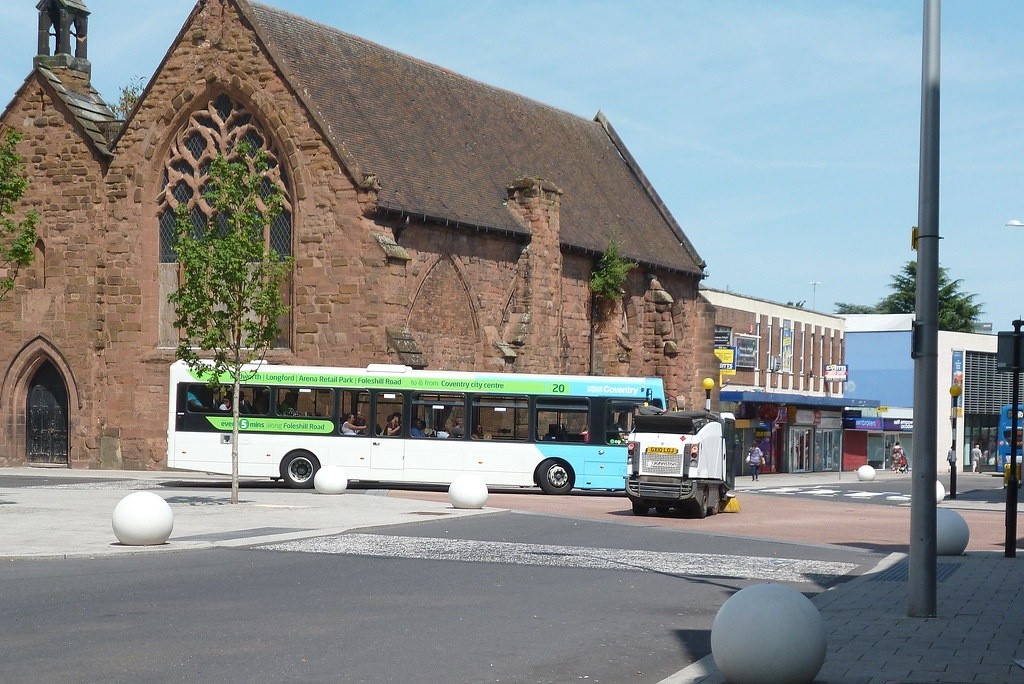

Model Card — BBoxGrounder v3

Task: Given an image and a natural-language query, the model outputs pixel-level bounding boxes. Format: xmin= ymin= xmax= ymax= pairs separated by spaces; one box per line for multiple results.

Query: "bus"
xmin=167 ymin=356 xmax=685 ymax=496
xmin=996 ymin=405 xmax=1023 ymax=473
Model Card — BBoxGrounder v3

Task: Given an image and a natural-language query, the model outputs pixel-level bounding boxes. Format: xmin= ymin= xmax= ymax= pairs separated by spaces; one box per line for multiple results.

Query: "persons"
xmin=218 ymin=398 xmax=232 ymax=410
xmin=892 ymin=441 xmax=903 ymax=474
xmin=223 ymin=386 xmax=232 ymax=402
xmin=471 ymin=423 xmax=483 ymax=439
xmin=188 ymin=392 xmax=203 ymax=409
xmin=340 ymin=411 xmax=368 ymax=435
xmin=451 ymin=422 xmax=462 ymax=439
xmin=239 ymin=390 xmax=259 ymax=414
xmin=748 ymin=443 xmax=763 ymax=481
xmin=411 ymin=418 xmax=427 ymax=437
xmin=383 ymin=411 xmax=402 ymax=436
xmin=971 ymin=445 xmax=984 ymax=474
xmin=436 ymin=428 xmax=450 ymax=438
xmin=279 ymin=392 xmax=301 ymax=416
xmin=946 ymin=446 xmax=952 ymax=472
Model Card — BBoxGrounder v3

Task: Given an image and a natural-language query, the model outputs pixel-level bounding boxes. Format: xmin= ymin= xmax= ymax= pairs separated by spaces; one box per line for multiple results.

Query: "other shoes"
xmin=752 ymin=479 xmax=754 ymax=481
xmin=756 ymin=478 xmax=758 ymax=480
xmin=948 ymin=469 xmax=951 ymax=473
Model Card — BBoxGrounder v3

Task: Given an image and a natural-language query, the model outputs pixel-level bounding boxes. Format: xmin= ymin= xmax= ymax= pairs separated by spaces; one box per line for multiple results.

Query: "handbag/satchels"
xmin=761 ymin=459 xmax=765 ymax=467
xmin=901 ymin=457 xmax=906 ymax=464
xmin=745 ymin=448 xmax=752 ymax=463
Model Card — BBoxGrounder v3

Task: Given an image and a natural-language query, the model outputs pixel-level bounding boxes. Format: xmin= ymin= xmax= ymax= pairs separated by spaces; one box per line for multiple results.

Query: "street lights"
xmin=949 ymin=385 xmax=963 ymax=499
xmin=702 ymin=377 xmax=715 ymax=413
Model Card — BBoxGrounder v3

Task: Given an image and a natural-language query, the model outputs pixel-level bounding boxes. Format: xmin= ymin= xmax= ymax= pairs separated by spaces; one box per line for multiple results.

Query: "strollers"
xmin=901 ymin=455 xmax=908 ymax=474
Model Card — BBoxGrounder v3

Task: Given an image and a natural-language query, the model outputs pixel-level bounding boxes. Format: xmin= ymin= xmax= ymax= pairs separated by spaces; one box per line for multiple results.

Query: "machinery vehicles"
xmin=624 ymin=405 xmax=740 ymax=519
